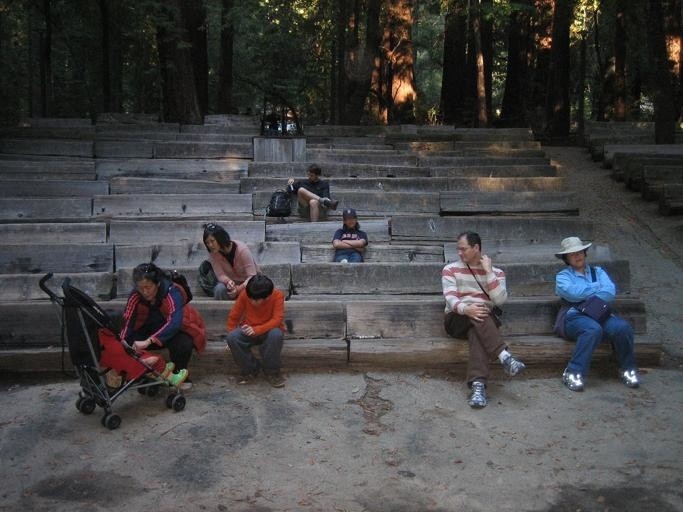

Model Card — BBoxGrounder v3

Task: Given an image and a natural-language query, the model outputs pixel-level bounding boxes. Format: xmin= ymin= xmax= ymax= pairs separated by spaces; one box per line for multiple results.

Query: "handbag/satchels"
xmin=577 ymin=295 xmax=611 ymax=323
xmin=491 ymin=305 xmax=502 ymax=326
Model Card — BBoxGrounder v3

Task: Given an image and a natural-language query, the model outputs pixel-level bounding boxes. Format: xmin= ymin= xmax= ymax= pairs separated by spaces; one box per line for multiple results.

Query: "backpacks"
xmin=197 ymin=260 xmax=218 ymax=297
xmin=266 ymin=189 xmax=292 ymax=217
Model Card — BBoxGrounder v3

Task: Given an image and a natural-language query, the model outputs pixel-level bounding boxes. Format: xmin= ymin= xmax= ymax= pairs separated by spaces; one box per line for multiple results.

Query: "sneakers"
xmin=469 ymin=381 xmax=487 ymax=407
xmin=619 ymin=366 xmax=638 ymax=387
xmin=563 ymin=368 xmax=583 ymax=391
xmin=237 ymin=373 xmax=286 ymax=388
xmin=500 ymin=354 xmax=527 ymax=378
xmin=160 ymin=362 xmax=192 ymax=391
xmin=324 ymin=198 xmax=338 ymax=210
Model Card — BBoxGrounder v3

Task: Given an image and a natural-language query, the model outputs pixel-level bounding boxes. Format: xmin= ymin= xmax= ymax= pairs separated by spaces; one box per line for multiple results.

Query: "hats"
xmin=554 ymin=236 xmax=592 ymax=258
xmin=343 ymin=209 xmax=356 ymax=216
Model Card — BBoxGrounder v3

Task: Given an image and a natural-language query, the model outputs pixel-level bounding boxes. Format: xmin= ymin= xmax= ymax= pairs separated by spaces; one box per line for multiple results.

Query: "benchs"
xmin=0 ymin=118 xmax=682 ymax=372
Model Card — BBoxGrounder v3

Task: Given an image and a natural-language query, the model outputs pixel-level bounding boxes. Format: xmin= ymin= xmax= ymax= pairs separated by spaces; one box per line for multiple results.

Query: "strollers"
xmin=34 ymin=270 xmax=191 ymax=430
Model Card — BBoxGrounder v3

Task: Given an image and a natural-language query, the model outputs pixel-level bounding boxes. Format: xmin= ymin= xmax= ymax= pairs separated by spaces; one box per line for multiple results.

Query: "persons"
xmin=226 ymin=274 xmax=287 ymax=387
xmin=115 ymin=262 xmax=196 ymax=369
xmin=287 ymin=165 xmax=337 ymax=222
xmin=201 ymin=222 xmax=258 ymax=300
xmin=332 ymin=209 xmax=367 ymax=263
xmin=107 ymin=337 xmax=189 ymax=387
xmin=555 ymin=237 xmax=638 ymax=391
xmin=441 ymin=232 xmax=526 ymax=407
